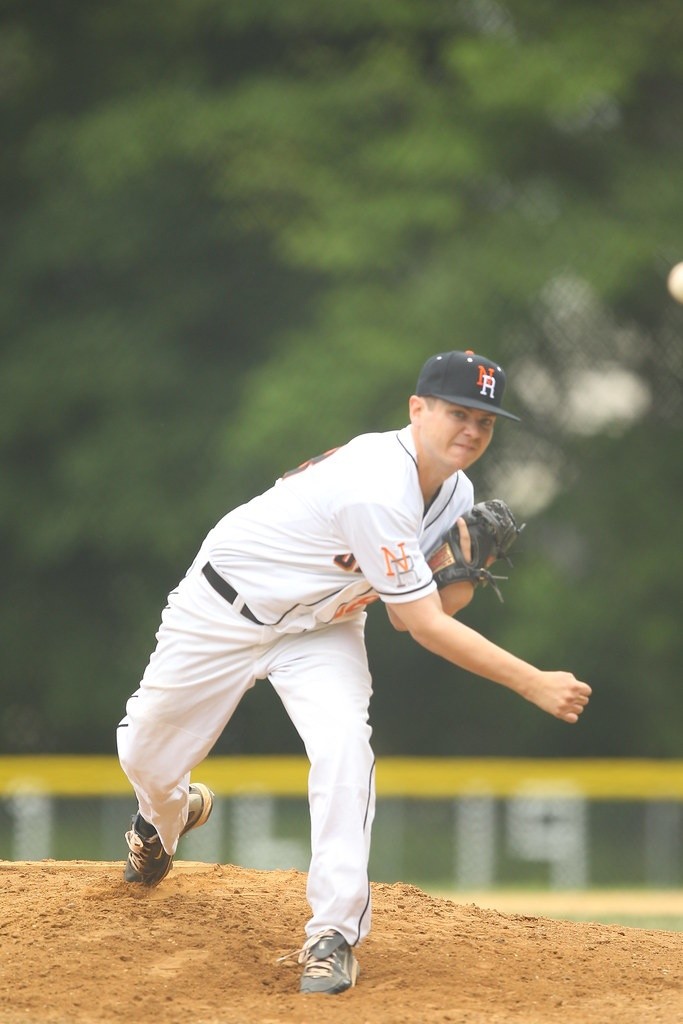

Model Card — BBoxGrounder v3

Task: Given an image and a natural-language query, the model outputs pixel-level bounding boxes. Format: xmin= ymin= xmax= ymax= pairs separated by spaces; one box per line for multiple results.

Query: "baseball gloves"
xmin=426 ymin=497 xmax=526 ymax=594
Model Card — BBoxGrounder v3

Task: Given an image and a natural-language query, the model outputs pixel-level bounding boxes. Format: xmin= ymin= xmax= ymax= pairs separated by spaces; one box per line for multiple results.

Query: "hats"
xmin=417 ymin=350 xmax=523 ymax=423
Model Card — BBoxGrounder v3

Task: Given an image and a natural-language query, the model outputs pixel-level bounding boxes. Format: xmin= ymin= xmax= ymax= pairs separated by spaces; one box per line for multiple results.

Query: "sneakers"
xmin=298 ymin=929 xmax=358 ymax=996
xmin=124 ymin=783 xmax=215 ymax=889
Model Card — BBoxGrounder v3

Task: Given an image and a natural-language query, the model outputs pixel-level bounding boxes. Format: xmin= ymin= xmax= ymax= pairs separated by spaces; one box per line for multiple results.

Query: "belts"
xmin=201 ymin=562 xmax=266 ymax=627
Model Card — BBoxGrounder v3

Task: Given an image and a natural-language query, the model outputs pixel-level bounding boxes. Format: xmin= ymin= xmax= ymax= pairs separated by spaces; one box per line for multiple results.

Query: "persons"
xmin=116 ymin=352 xmax=592 ymax=994
